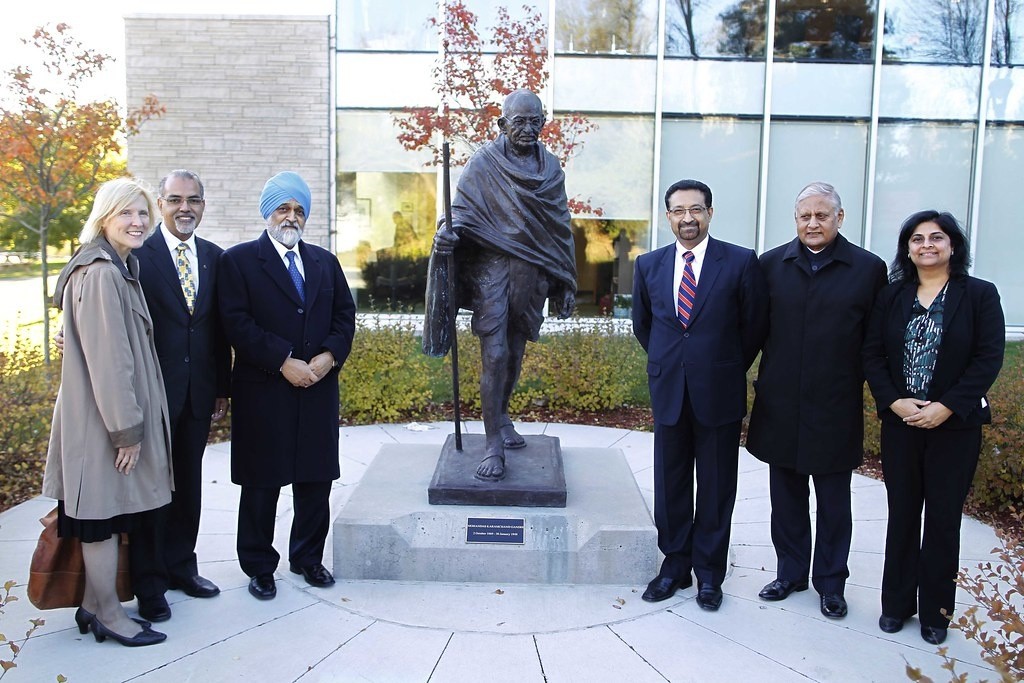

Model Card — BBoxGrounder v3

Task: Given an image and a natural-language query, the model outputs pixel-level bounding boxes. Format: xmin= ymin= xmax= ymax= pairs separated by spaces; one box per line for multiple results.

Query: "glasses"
xmin=161 ymin=197 xmax=203 ymax=205
xmin=669 ymin=207 xmax=709 ymax=215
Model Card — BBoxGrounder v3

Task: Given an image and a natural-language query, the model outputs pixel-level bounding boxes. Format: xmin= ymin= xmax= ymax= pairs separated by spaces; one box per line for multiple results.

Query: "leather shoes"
xmin=139 ymin=593 xmax=171 ymax=621
xmin=879 ymin=614 xmax=905 ymax=632
xmin=921 ymin=625 xmax=947 ymax=644
xmin=290 ymin=562 xmax=335 ymax=587
xmin=697 ymin=580 xmax=723 ymax=610
xmin=170 ymin=574 xmax=220 ymax=598
xmin=249 ymin=573 xmax=276 ymax=599
xmin=641 ymin=572 xmax=692 ymax=602
xmin=759 ymin=579 xmax=808 ymax=601
xmin=821 ymin=592 xmax=847 ymax=617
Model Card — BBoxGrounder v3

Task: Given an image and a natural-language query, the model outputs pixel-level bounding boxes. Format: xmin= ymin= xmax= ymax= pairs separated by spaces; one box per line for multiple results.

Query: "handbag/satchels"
xmin=27 ymin=507 xmax=135 ymax=610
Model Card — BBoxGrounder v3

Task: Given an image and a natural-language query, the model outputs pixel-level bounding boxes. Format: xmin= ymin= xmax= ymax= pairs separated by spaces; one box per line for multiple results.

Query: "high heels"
xmin=75 ymin=605 xmax=151 ymax=634
xmin=91 ymin=614 xmax=167 ymax=646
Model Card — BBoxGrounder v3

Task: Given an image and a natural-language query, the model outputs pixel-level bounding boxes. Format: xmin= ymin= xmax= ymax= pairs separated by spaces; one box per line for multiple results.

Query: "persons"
xmin=426 ymin=89 xmax=577 ymax=480
xmin=632 ymin=180 xmax=759 ymax=610
xmin=216 ymin=171 xmax=354 ymax=599
xmin=860 ymin=211 xmax=1006 ymax=643
xmin=43 ymin=176 xmax=168 ymax=647
xmin=744 ymin=182 xmax=889 ymax=620
xmin=393 ymin=211 xmax=418 ymax=246
xmin=54 ymin=167 xmax=233 ymax=622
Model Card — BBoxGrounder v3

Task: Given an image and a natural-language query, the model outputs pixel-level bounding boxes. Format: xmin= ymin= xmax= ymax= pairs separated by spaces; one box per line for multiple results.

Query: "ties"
xmin=678 ymin=251 xmax=696 ymax=330
xmin=285 ymin=251 xmax=305 ymax=303
xmin=177 ymin=243 xmax=196 ymax=315
